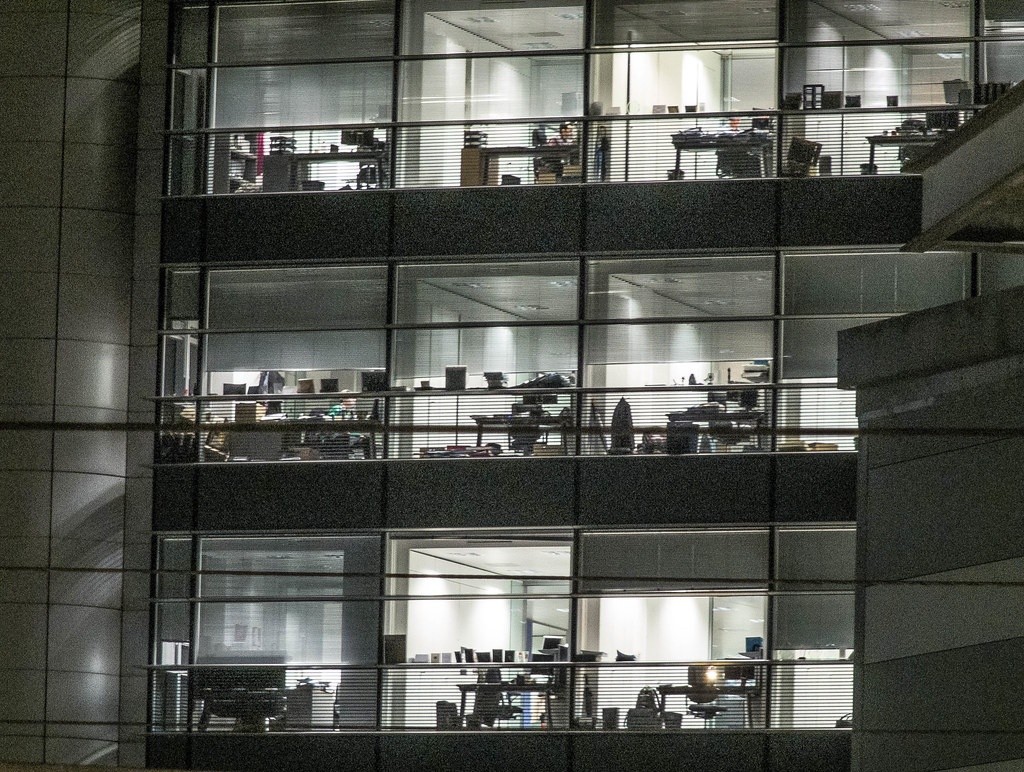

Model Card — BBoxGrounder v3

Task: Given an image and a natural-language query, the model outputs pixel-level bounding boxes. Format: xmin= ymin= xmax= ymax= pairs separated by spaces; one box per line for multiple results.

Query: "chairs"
xmin=531 ymin=128 xmax=562 ymax=177
xmin=707 ymin=392 xmax=750 ymax=453
xmin=304 ymin=410 xmax=350 ymax=460
xmin=508 ymin=404 xmax=551 ymax=457
xmin=686 ymin=666 xmax=727 ymax=728
xmin=483 ymin=669 xmax=524 ymax=728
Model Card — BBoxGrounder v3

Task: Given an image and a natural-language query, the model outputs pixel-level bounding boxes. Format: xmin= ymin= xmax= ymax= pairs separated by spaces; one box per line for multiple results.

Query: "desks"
xmin=670 ymin=134 xmax=773 ymax=180
xmin=280 ymin=417 xmax=376 ymax=460
xmin=864 ymin=134 xmax=941 ymax=175
xmin=197 ymin=687 xmax=290 ymax=731
xmin=289 ymin=151 xmax=386 ymax=190
xmin=478 ymin=145 xmax=580 ymax=185
xmin=657 ymin=685 xmax=761 ymax=728
xmin=456 ymin=683 xmax=569 ymax=728
xmin=665 ymin=412 xmax=769 ymax=451
xmin=469 ymin=414 xmax=574 ymax=455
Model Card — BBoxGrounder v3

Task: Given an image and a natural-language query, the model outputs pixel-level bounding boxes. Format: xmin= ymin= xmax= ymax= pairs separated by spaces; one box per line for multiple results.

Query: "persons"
xmin=547 ymin=122 xmax=577 ymax=146
xmin=327 ymin=389 xmax=370 ymax=462
xmin=719 ymin=116 xmax=745 ymax=135
